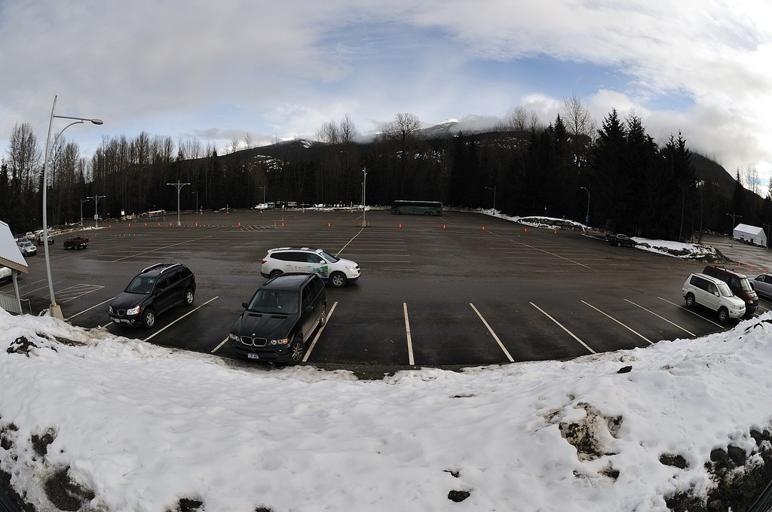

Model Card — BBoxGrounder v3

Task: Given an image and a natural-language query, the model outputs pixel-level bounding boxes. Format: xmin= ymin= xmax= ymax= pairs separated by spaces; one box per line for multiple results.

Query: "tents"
xmin=733 ymin=223 xmax=767 ymax=249
xmin=660 ymin=222 xmax=698 ymax=242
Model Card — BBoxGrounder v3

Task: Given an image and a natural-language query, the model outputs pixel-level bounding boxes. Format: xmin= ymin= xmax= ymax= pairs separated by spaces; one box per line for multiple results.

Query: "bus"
xmin=391 ymin=200 xmax=443 ymax=216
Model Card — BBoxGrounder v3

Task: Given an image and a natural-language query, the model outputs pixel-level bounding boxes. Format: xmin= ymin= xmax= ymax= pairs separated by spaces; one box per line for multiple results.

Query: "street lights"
xmin=166 ymin=180 xmax=191 ymax=225
xmin=580 ymin=187 xmax=591 ymax=226
xmin=191 ymin=192 xmax=198 ymax=212
xmin=484 ymin=185 xmax=496 ymax=215
xmin=80 ymin=194 xmax=106 ymax=227
xmin=42 ymin=95 xmax=103 ymax=304
xmin=359 ymin=166 xmax=369 ymax=227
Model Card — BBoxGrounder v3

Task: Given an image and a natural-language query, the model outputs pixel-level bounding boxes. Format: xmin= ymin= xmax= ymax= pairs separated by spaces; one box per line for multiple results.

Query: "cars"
xmin=255 ymin=203 xmax=267 ymax=210
xmin=17 ymin=230 xmax=54 ymax=256
xmin=228 ymin=246 xmax=361 ymax=366
xmin=107 ymin=263 xmax=196 ymax=329
xmin=681 ymin=264 xmax=772 ymax=323
xmin=64 ymin=237 xmax=88 ymax=250
xmin=607 ymin=234 xmax=635 ymax=247
